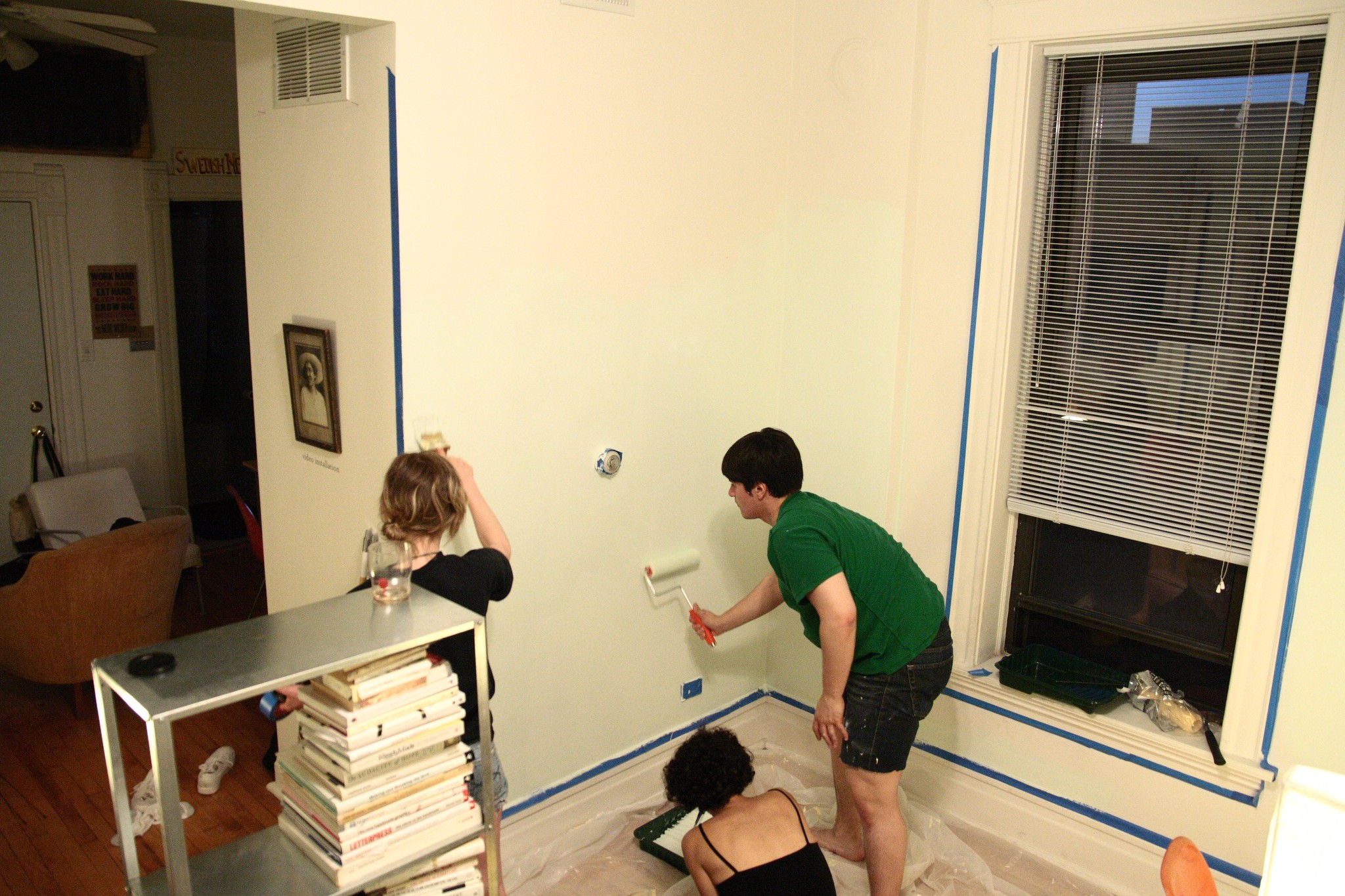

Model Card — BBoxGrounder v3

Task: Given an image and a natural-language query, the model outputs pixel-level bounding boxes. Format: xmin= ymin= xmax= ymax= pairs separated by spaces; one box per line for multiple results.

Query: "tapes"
xmin=259 ymin=691 xmax=281 ymax=720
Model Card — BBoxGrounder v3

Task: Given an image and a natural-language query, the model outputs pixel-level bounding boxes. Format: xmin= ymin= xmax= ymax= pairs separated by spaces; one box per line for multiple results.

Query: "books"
xmin=266 ymin=642 xmax=485 ymax=896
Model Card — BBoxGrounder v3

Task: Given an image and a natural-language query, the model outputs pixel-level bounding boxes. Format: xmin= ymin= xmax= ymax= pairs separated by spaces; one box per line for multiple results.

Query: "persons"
xmin=689 ymin=427 xmax=954 ymax=896
xmin=663 ymin=726 xmax=837 ymax=896
xmin=277 ymin=447 xmax=514 ymax=896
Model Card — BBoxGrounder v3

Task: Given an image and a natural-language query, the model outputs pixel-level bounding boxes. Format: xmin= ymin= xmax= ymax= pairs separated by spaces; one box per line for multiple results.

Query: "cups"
xmin=368 ymin=539 xmax=413 ymax=603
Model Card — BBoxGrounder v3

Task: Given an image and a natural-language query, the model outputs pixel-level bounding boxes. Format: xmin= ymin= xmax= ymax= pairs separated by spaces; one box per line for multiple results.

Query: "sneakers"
xmin=132 ymin=768 xmax=157 ymax=810
xmin=197 ymin=745 xmax=236 ymax=794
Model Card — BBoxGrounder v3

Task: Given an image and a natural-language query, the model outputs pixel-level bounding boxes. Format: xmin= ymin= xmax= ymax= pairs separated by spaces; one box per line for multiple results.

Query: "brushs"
xmin=643 ymin=549 xmax=717 ymax=648
xmin=416 ymin=431 xmax=450 ymax=458
xmin=1137 ymin=688 xmax=1226 ymax=766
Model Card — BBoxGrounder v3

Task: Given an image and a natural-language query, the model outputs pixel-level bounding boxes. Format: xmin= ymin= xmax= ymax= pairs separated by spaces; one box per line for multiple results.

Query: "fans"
xmin=0 ymin=0 xmax=158 ymax=71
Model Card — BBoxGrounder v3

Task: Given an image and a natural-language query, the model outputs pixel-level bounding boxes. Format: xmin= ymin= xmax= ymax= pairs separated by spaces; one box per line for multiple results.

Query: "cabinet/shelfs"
xmin=91 ymin=582 xmax=499 ymax=896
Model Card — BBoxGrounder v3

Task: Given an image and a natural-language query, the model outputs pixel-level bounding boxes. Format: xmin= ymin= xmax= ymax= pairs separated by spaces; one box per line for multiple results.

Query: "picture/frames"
xmin=282 ymin=323 xmax=342 ymax=454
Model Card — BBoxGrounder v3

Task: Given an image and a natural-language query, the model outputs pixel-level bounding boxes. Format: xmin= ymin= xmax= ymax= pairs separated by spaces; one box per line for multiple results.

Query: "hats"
xmin=299 ymin=353 xmax=323 ymax=385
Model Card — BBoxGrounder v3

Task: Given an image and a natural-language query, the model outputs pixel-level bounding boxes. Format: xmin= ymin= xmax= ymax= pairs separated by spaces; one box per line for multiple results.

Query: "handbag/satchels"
xmin=8 ymin=494 xmax=44 ymax=552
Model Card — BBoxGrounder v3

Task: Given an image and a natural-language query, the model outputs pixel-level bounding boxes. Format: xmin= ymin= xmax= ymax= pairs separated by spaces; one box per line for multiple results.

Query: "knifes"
xmin=1199 ymin=712 xmax=1226 ymax=766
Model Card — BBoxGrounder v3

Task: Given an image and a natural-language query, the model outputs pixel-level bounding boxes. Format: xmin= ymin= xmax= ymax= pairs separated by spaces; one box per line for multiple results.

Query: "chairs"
xmin=226 ymin=483 xmax=265 ymax=621
xmin=0 ymin=513 xmax=192 ymax=718
xmin=33 ymin=505 xmax=204 ymax=613
